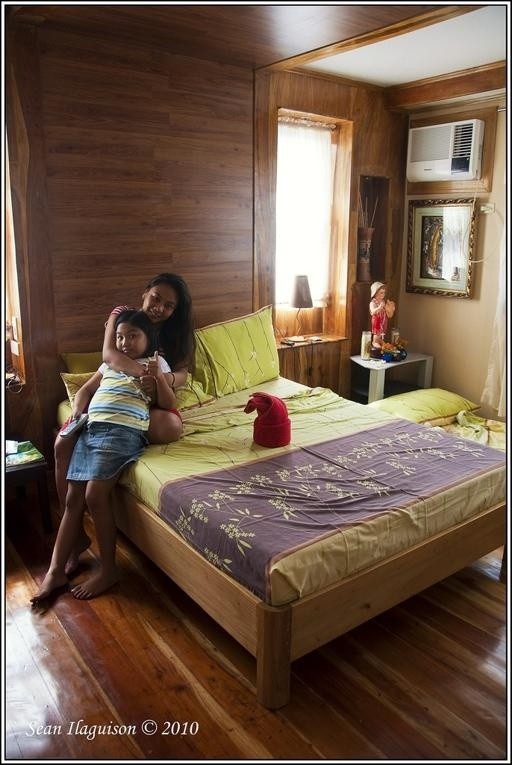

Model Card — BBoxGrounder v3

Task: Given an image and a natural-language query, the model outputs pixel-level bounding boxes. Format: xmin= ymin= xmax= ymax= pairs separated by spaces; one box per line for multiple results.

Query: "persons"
xmin=54 ymin=272 xmax=197 ymax=579
xmin=30 ymin=309 xmax=179 ymax=610
xmin=367 ymin=279 xmax=407 ymax=363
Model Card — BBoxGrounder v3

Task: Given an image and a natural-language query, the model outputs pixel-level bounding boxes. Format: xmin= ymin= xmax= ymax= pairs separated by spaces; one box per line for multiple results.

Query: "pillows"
xmin=56 ymin=349 xmax=104 ymax=374
xmin=59 ymin=370 xmax=217 ymax=412
xmin=193 ymin=303 xmax=284 ymax=400
xmin=367 ymin=386 xmax=483 ymax=427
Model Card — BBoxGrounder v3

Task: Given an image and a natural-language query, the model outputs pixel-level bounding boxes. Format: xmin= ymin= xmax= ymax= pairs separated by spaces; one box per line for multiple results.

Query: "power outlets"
xmin=479 ymin=203 xmax=495 ymax=215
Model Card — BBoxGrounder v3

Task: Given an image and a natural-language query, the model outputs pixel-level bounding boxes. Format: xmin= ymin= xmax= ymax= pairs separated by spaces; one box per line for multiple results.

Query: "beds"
xmin=56 ymin=362 xmax=507 ymax=712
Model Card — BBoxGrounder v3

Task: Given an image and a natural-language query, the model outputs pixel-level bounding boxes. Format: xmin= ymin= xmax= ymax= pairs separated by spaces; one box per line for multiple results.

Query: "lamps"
xmin=284 ymin=274 xmax=314 ymax=342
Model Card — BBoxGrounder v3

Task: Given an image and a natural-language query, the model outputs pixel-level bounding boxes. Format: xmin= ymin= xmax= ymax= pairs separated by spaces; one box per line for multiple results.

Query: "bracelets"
xmin=168 ymin=371 xmax=176 ymax=385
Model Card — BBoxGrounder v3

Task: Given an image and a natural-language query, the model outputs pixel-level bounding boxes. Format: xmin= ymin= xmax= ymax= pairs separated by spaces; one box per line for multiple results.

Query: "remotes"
xmin=281 ymin=340 xmax=295 ymax=345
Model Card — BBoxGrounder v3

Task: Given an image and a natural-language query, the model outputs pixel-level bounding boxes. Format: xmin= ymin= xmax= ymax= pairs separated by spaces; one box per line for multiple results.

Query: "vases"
xmin=356 ymin=225 xmax=377 ymax=282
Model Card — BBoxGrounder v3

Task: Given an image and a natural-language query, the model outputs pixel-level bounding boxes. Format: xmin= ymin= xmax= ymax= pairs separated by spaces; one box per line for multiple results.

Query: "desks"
xmin=6 ymin=439 xmax=54 ymax=535
xmin=276 ymin=335 xmax=350 ymax=393
xmin=349 ymin=352 xmax=435 ymax=405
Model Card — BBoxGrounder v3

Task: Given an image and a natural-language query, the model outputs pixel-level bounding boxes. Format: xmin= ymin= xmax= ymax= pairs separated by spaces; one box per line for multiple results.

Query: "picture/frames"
xmin=404 ymin=196 xmax=479 ymax=300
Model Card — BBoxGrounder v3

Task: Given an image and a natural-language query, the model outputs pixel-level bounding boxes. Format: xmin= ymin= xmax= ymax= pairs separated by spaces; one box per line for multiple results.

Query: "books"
xmin=6 ymin=440 xmax=48 ymax=474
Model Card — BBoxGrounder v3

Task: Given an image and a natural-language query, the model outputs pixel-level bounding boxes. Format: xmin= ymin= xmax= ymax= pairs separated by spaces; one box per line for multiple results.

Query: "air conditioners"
xmin=405 ymin=117 xmax=486 ymax=185
